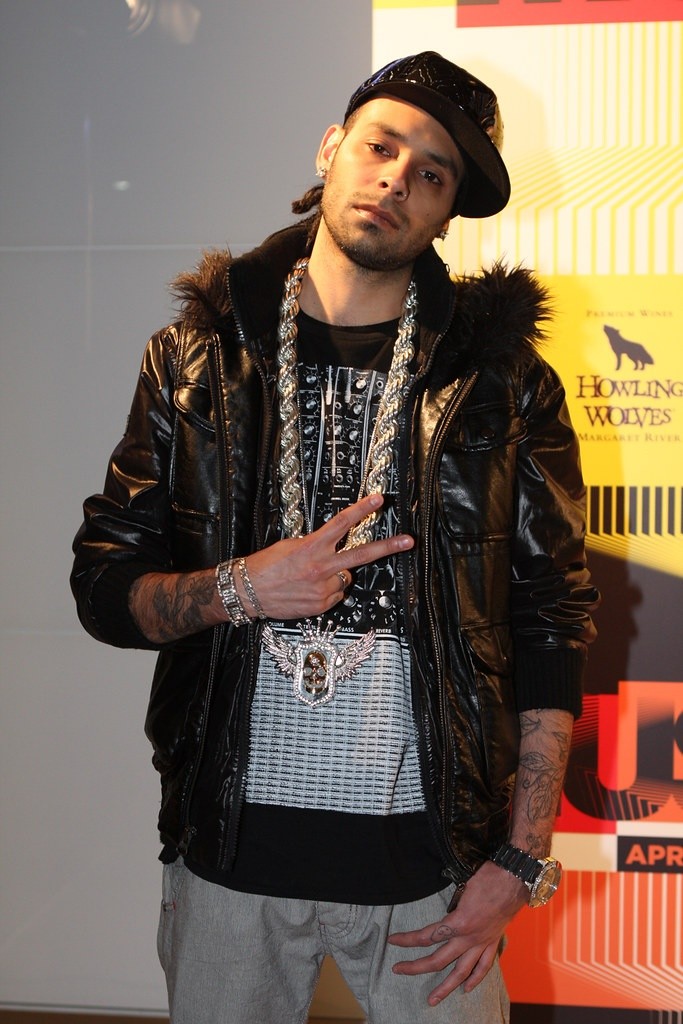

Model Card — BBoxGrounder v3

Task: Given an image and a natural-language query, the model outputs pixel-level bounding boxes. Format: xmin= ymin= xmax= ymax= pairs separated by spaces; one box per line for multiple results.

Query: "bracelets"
xmin=214 ymin=558 xmax=256 ymax=627
xmin=238 ymin=556 xmax=265 ymax=620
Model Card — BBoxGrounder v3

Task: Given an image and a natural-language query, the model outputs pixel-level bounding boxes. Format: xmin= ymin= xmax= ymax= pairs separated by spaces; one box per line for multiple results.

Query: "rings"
xmin=336 ymin=572 xmax=348 ymax=592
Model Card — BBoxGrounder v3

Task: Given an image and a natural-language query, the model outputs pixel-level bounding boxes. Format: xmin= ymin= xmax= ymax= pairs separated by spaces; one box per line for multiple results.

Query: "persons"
xmin=69 ymin=52 xmax=600 ymax=1022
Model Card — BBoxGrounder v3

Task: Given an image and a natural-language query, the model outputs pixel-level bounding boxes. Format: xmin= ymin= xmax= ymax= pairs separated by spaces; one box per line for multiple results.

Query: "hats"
xmin=343 ymin=51 xmax=511 ymax=219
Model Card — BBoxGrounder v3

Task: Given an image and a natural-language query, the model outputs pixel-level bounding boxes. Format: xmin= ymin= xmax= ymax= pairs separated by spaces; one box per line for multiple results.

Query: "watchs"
xmin=489 ymin=843 xmax=562 ymax=907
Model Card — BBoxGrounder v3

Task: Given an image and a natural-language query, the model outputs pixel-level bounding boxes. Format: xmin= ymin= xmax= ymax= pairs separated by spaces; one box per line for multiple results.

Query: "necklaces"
xmin=278 ymin=257 xmax=419 ymax=555
